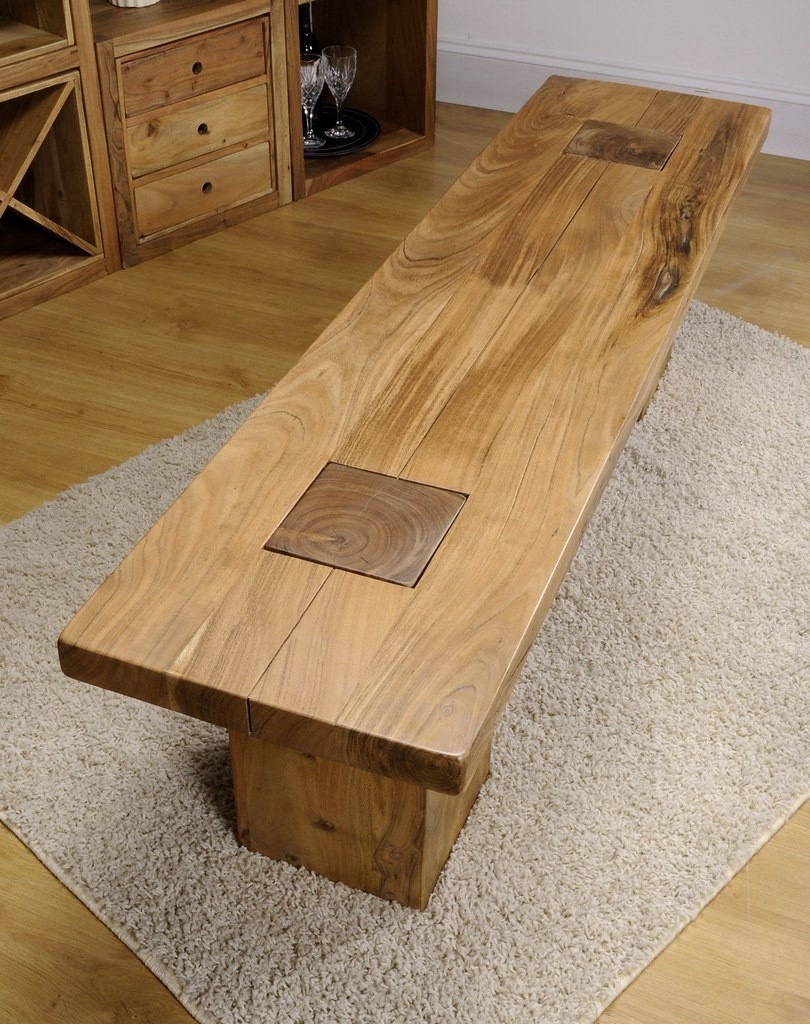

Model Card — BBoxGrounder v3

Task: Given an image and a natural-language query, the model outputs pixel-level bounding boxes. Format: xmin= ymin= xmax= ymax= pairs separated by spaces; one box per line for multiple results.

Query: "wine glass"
xmin=322 ymin=45 xmax=357 ymax=138
xmin=301 ymin=54 xmax=324 ymax=148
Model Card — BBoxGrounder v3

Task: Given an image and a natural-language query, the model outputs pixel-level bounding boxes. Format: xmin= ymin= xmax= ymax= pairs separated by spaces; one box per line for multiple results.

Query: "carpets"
xmin=1 ymin=295 xmax=810 ymax=1023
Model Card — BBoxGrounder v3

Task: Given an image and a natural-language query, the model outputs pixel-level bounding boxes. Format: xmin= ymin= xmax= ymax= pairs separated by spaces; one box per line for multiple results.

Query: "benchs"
xmin=55 ymin=73 xmax=772 ymax=910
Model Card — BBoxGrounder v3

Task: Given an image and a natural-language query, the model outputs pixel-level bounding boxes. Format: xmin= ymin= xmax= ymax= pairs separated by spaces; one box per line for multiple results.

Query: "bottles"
xmin=299 ymin=2 xmax=322 ymax=54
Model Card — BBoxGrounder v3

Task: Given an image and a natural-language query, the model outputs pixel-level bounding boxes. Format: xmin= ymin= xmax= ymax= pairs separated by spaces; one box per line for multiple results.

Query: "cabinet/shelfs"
xmin=0 ymin=1 xmax=438 ymax=319
